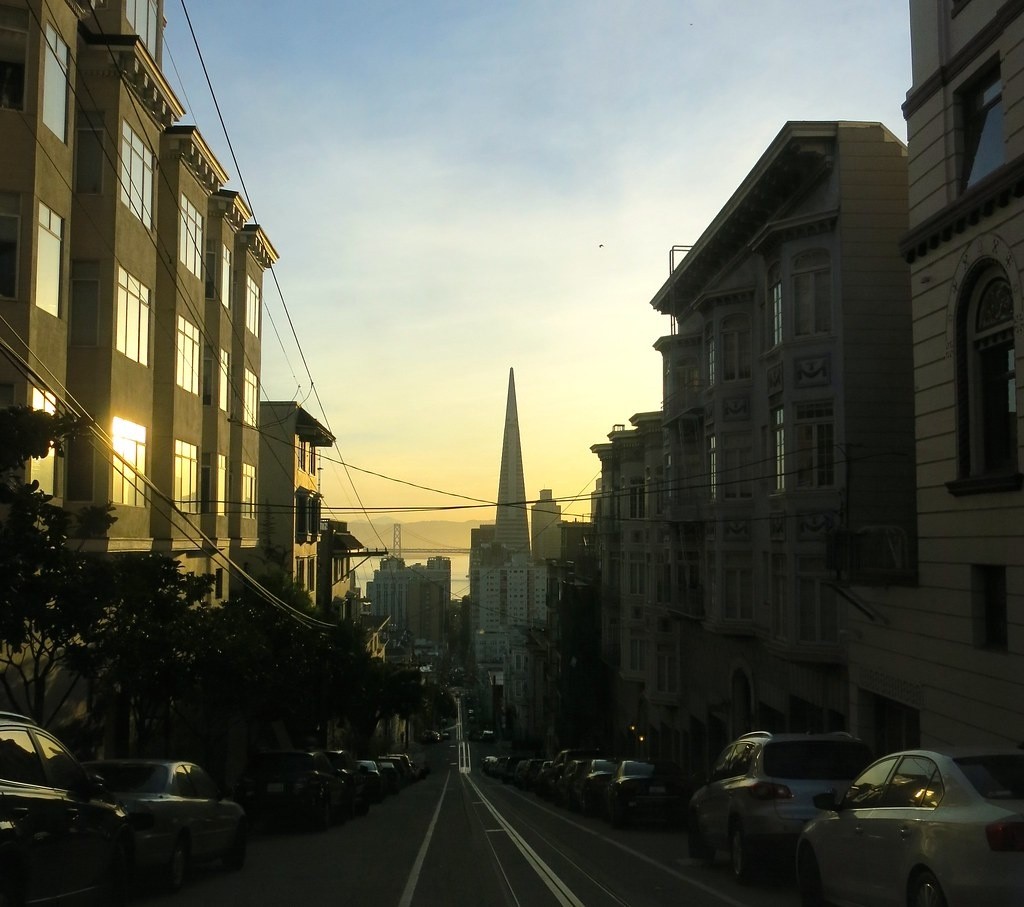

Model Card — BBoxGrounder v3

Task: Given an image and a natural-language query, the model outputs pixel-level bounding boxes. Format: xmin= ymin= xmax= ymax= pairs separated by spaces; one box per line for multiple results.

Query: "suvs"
xmin=326 ymin=751 xmax=364 ymax=808
xmin=0 ymin=711 xmax=129 ymax=907
xmin=689 ymin=731 xmax=883 ymax=885
xmin=479 ymin=731 xmax=495 ymax=742
xmin=541 ymin=749 xmax=595 ymax=796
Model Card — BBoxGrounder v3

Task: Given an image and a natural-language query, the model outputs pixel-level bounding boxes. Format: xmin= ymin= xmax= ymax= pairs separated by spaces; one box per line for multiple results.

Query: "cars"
xmin=484 ymin=753 xmax=552 ymax=788
xmin=797 ymin=750 xmax=1024 ymax=907
xmin=357 ymin=753 xmax=417 ymax=802
xmin=421 ymin=730 xmax=437 ymax=742
xmin=607 ymin=760 xmax=690 ymax=827
xmin=443 ymin=730 xmax=450 ymax=736
xmin=556 ymin=760 xmax=588 ymax=805
xmin=577 ymin=760 xmax=622 ymax=816
xmin=79 ymin=760 xmax=247 ymax=894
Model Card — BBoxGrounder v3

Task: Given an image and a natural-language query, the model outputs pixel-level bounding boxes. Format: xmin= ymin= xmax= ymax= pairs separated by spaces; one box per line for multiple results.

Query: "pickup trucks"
xmin=234 ymin=747 xmax=351 ymax=831
